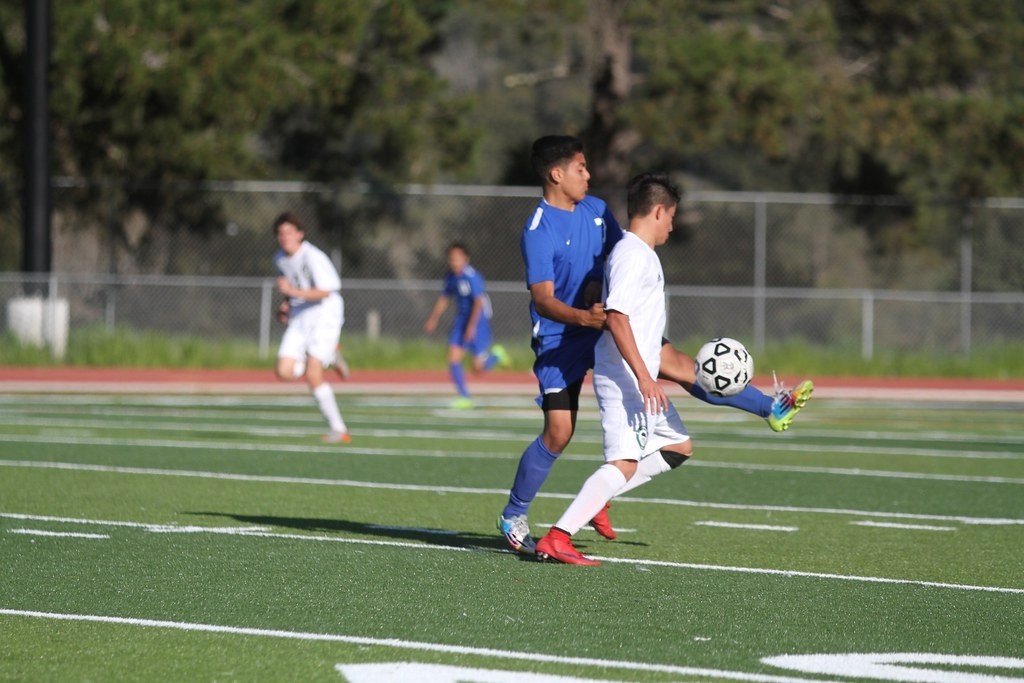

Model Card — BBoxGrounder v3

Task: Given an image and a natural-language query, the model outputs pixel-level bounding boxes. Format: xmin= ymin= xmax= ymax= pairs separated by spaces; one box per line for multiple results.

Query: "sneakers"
xmin=589 ymin=503 xmax=616 ymax=540
xmin=495 ymin=513 xmax=537 ymax=556
xmin=765 ymin=371 xmax=814 ymax=432
xmin=535 ymin=526 xmax=602 ymax=566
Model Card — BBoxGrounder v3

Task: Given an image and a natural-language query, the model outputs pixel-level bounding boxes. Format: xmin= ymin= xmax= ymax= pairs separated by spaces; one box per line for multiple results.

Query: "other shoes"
xmin=448 ymin=397 xmax=472 ymax=408
xmin=331 ymin=346 xmax=349 ymax=380
xmin=491 ymin=345 xmax=512 ymax=370
xmin=319 ymin=432 xmax=354 ymax=444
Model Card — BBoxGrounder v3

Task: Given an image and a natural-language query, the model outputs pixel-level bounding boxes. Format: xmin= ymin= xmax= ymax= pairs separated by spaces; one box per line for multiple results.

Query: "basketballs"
xmin=694 ymin=337 xmax=755 ymax=397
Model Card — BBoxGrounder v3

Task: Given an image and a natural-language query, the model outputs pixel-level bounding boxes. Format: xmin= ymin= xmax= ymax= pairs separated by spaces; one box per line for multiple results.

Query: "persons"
xmin=272 ymin=212 xmax=352 ymax=445
xmin=535 ymin=171 xmax=693 ymax=565
xmin=425 ymin=244 xmax=513 ymax=409
xmin=495 ymin=135 xmax=814 ymax=555
